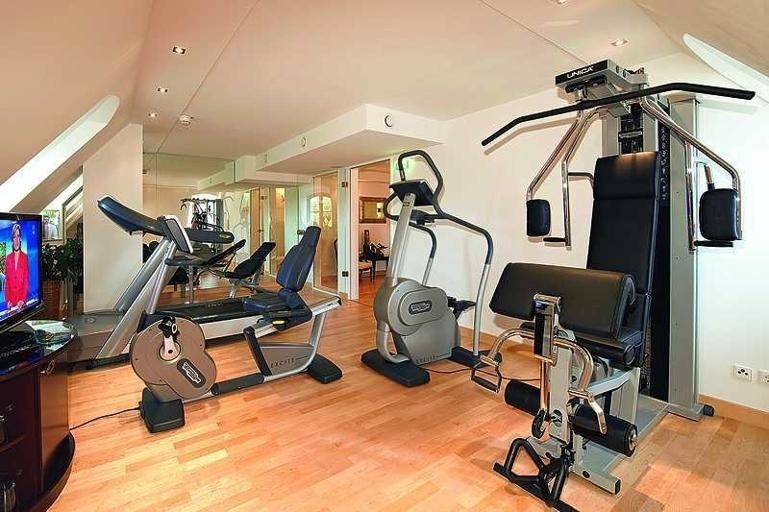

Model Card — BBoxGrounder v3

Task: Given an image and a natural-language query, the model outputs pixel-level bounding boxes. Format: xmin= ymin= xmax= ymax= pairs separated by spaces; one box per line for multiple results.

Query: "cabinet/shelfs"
xmin=0 ymin=317 xmax=80 ymax=511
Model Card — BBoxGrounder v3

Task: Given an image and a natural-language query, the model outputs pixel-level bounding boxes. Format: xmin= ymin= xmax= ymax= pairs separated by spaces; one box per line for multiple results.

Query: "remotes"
xmin=0 ymin=343 xmax=40 ymax=362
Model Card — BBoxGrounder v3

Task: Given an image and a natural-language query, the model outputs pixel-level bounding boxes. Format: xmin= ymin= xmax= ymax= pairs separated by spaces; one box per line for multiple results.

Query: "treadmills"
xmin=64 ymin=195 xmax=264 ymax=375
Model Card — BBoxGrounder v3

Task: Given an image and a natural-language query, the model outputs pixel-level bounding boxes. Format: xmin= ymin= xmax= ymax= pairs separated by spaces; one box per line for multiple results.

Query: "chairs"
xmin=242 ymin=225 xmax=323 ymax=325
xmin=334 ymin=236 xmax=374 ymax=283
xmin=214 ymin=240 xmax=277 ymax=298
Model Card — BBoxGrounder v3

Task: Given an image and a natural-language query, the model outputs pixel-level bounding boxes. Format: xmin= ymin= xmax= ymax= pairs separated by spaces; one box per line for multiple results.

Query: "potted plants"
xmin=37 ymin=237 xmax=82 ymax=319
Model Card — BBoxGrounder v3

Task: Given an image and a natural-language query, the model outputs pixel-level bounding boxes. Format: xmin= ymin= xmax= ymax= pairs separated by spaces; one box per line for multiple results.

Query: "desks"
xmin=359 ymin=253 xmax=391 ymax=283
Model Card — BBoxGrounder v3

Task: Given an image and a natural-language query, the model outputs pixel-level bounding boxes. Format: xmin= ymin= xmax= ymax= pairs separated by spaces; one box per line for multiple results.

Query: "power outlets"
xmin=756 ymin=368 xmax=768 ymax=385
xmin=733 ymin=363 xmax=753 ymax=381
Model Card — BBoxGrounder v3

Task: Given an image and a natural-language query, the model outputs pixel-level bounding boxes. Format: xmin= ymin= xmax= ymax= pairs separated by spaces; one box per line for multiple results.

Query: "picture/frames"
xmin=40 ymin=207 xmax=65 ymax=245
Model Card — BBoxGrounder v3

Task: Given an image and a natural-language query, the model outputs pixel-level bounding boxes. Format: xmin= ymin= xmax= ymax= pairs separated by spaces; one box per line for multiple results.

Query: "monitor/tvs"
xmin=0 ymin=213 xmax=44 ymax=350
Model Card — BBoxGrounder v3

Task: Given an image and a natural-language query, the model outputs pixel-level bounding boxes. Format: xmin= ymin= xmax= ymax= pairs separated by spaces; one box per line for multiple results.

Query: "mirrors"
xmin=141 ymin=149 xmax=263 ymax=310
xmin=359 ymin=195 xmax=388 ymax=224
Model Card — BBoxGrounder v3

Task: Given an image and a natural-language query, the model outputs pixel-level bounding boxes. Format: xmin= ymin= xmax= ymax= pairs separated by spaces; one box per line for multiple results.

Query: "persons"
xmin=4 ymin=224 xmax=30 ymax=310
